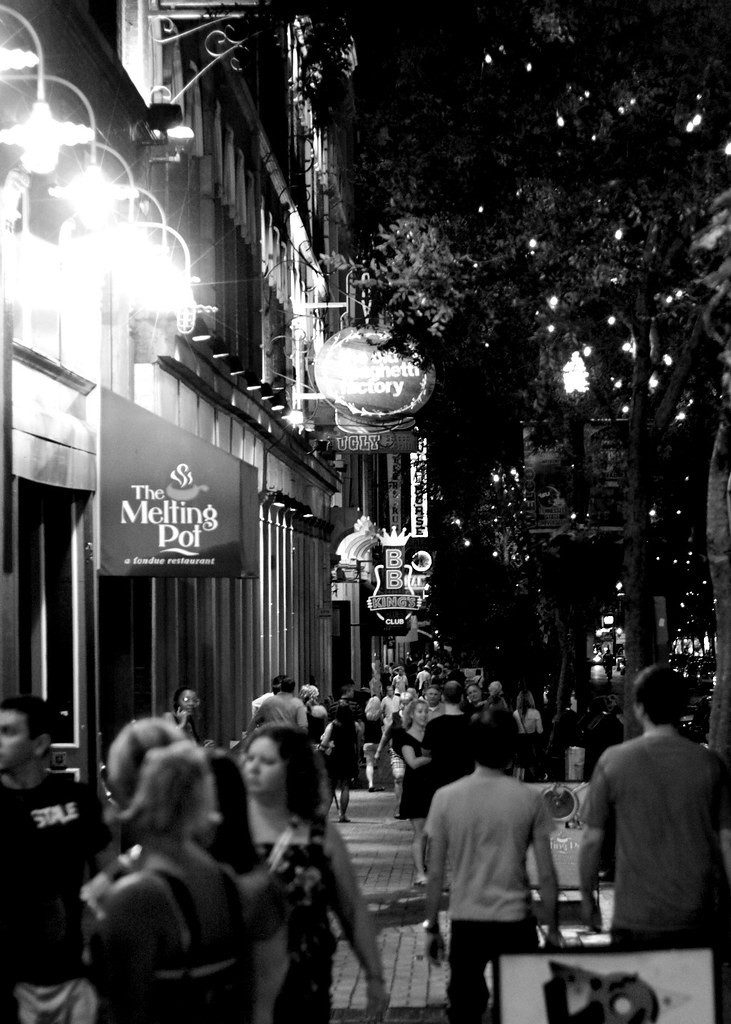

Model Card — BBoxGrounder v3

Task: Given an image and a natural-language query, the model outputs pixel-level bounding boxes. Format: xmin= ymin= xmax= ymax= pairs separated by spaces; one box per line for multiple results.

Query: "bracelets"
xmin=423 ymin=921 xmax=438 ymax=931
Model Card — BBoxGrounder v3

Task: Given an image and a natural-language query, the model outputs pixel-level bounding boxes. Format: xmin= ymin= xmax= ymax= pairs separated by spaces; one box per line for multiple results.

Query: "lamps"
xmin=0 ymin=74 xmax=140 ymax=231
xmin=0 ymin=3 xmax=96 ymax=174
xmin=143 ymin=221 xmax=220 ymax=334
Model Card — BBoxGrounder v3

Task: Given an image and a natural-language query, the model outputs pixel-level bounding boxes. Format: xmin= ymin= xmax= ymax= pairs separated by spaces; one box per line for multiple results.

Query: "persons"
xmin=382 ymin=647 xmax=508 ymax=721
xmin=247 ymin=677 xmax=309 ymax=735
xmin=382 ymin=664 xmax=391 ymax=690
xmin=381 ymin=686 xmax=401 ymax=725
xmin=90 ymin=718 xmax=257 ymax=1024
xmin=0 ymin=696 xmax=117 ymax=1023
xmin=578 ymin=662 xmax=731 ymax=955
xmin=424 ymin=684 xmax=446 ymax=718
xmin=321 ymin=700 xmax=362 ymax=823
xmin=340 ymin=685 xmax=354 ymax=702
xmin=241 ymin=720 xmax=390 ymax=1024
xmin=363 ymin=696 xmax=385 ymax=793
xmin=513 ymin=690 xmax=544 ymax=780
xmin=392 ymin=666 xmax=409 ymax=694
xmin=421 ymin=680 xmax=487 ymax=794
xmin=252 ymin=675 xmax=287 ymax=719
xmin=163 ymin=688 xmax=216 ymax=749
xmin=393 ymin=700 xmax=433 ymax=884
xmin=300 ymin=683 xmax=333 ymax=817
xmin=423 ymin=708 xmax=564 ymax=1022
